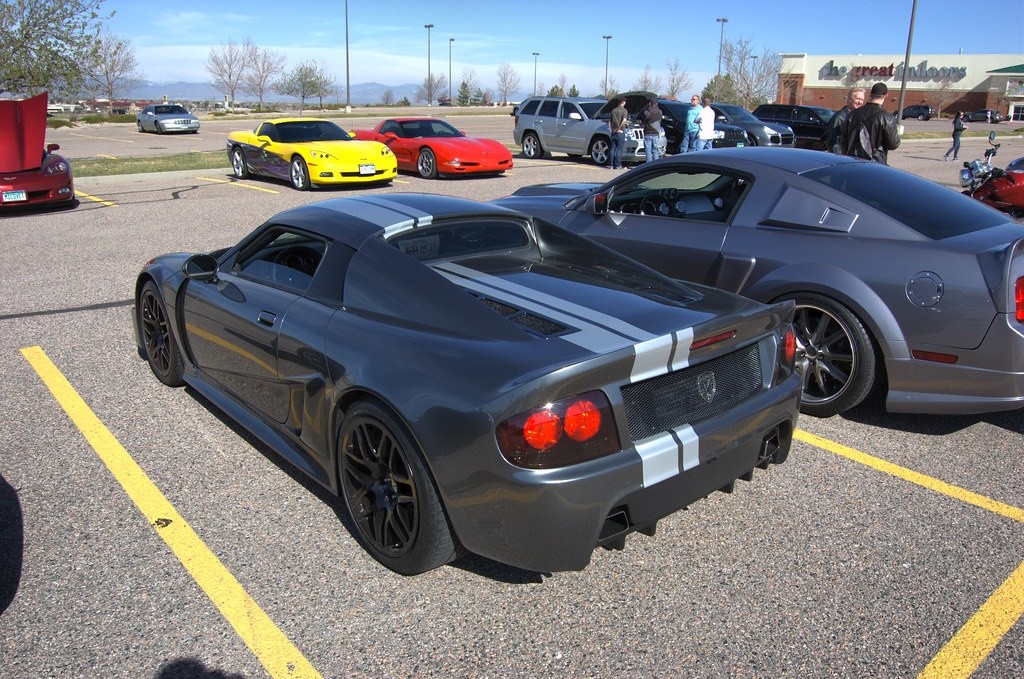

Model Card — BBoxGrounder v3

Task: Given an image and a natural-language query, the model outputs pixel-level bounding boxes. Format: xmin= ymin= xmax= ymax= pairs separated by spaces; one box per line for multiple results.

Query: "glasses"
xmin=691 ymin=98 xmax=699 ymax=100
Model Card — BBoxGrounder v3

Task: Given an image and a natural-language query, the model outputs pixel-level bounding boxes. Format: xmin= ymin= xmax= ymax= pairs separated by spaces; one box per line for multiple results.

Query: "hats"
xmin=871 ymin=82 xmax=887 ymax=95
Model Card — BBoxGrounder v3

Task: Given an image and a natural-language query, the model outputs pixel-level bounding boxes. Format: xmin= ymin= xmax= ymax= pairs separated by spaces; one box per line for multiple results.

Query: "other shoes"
xmin=954 ymin=158 xmax=959 ymax=161
xmin=610 ymin=165 xmax=627 ymax=169
xmin=943 ymin=155 xmax=949 ymax=162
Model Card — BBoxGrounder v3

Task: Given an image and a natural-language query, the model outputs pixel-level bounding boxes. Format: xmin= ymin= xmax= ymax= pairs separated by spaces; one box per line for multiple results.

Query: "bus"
xmin=701 ymin=102 xmax=797 ymax=148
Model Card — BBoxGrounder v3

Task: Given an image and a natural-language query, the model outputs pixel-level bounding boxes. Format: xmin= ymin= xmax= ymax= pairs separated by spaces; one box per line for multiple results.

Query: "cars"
xmin=961 ymin=108 xmax=1004 ymax=123
xmin=136 ymin=104 xmax=201 ymax=134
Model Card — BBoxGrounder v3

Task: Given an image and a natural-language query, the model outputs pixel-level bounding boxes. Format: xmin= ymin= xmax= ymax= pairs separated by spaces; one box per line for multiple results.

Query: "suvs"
xmin=513 ymin=96 xmax=670 ymax=165
xmin=751 ymin=103 xmax=838 ymax=150
xmin=893 ymin=105 xmax=936 ymax=122
xmin=656 ymin=100 xmax=749 ymax=153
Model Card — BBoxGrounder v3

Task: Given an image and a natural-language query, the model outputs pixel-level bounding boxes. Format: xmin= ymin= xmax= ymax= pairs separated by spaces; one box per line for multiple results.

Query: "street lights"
xmin=424 ymin=24 xmax=435 ymax=106
xmin=532 ymin=52 xmax=540 ymax=96
xmin=748 ymin=56 xmax=760 ymax=106
xmin=716 ymin=17 xmax=730 ymax=74
xmin=602 ymin=35 xmax=612 ymax=96
xmin=449 ymin=37 xmax=455 ymax=103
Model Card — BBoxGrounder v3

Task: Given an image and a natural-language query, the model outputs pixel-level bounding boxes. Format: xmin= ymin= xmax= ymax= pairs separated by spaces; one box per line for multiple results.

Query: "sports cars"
xmin=0 ymin=92 xmax=76 ymax=210
xmin=130 ymin=190 xmax=812 ymax=575
xmin=227 ymin=117 xmax=400 ymax=192
xmin=350 ymin=117 xmax=514 ymax=178
xmin=489 ymin=143 xmax=1023 ymax=418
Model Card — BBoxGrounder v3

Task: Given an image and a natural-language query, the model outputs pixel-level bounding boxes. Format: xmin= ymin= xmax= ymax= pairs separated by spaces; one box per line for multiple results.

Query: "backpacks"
xmin=847 ymin=109 xmax=883 ymax=159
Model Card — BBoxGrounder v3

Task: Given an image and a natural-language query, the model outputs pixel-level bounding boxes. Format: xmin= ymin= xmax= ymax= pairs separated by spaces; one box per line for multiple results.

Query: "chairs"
xmin=386 ymin=126 xmax=401 ymax=138
xmin=800 ymin=113 xmax=809 ymax=121
xmin=416 ymin=126 xmax=436 ymax=138
xmin=395 ymin=226 xmax=454 ymax=262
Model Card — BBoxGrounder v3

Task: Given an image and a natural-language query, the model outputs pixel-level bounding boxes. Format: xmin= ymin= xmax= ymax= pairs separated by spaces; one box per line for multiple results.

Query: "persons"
xmin=607 ymin=98 xmax=628 ymax=169
xmin=841 ymin=83 xmax=900 ymax=165
xmin=693 ymin=97 xmax=716 ymax=149
xmin=641 ymin=99 xmax=663 ymax=163
xmin=679 ymin=95 xmax=704 ymax=153
xmin=944 ymin=111 xmax=968 ymax=161
xmin=822 ymin=88 xmax=866 ymax=151
xmin=986 ymin=110 xmax=992 ymax=124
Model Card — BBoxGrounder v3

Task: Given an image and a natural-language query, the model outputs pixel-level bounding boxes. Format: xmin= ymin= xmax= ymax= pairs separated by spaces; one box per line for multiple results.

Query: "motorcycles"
xmin=959 ymin=130 xmax=1024 ymax=219
